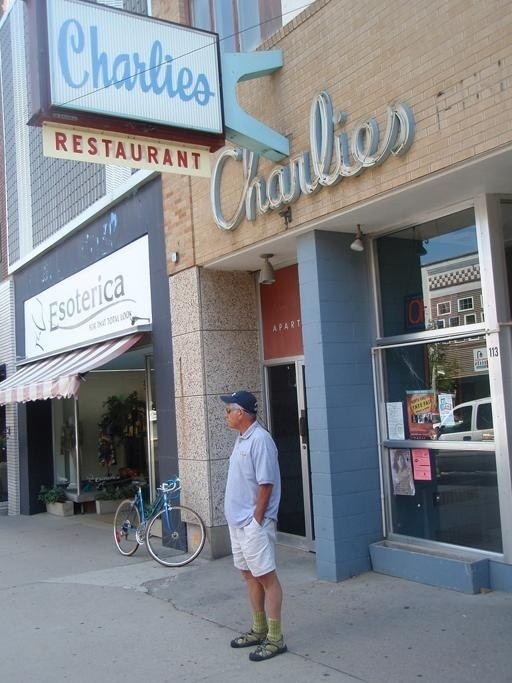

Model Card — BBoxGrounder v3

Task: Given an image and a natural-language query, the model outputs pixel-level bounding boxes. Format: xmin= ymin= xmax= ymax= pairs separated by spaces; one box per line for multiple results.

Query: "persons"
xmin=219 ymin=391 xmax=287 ymax=662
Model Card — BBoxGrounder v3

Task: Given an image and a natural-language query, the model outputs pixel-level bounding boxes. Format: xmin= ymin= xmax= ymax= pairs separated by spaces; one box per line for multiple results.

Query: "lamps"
xmin=256 ymin=249 xmax=278 ymax=284
xmin=350 ymin=221 xmax=366 ymax=251
xmin=130 ymin=315 xmax=151 ymax=326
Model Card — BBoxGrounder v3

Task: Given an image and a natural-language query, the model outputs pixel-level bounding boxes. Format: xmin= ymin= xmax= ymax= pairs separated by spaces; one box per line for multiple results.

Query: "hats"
xmin=220 ymin=391 xmax=258 ymax=413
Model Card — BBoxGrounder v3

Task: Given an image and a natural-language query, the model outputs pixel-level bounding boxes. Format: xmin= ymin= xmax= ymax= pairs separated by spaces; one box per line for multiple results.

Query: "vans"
xmin=432 ymin=396 xmax=496 ymax=473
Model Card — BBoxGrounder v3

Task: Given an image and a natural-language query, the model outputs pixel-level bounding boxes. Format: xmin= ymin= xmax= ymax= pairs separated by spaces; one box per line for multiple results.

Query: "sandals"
xmin=231 ymin=628 xmax=268 ymax=648
xmin=249 ymin=634 xmax=287 ymax=661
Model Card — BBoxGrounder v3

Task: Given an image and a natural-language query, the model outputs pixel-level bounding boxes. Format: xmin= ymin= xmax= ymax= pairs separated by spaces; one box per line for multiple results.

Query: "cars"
xmin=431 ymin=412 xmax=462 ymax=430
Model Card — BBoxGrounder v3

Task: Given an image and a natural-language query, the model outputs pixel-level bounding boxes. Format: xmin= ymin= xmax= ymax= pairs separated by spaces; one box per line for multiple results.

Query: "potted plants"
xmin=91 ymin=482 xmax=138 ymax=513
xmin=36 ymin=482 xmax=73 ymax=517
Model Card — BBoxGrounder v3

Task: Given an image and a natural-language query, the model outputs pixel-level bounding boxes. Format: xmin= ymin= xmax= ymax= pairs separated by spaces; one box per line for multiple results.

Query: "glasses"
xmin=225 ymin=408 xmax=240 ymax=413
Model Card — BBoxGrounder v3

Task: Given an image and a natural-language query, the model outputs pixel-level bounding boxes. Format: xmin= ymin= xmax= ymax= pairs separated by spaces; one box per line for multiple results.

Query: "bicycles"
xmin=114 ymin=476 xmax=208 ymax=569
